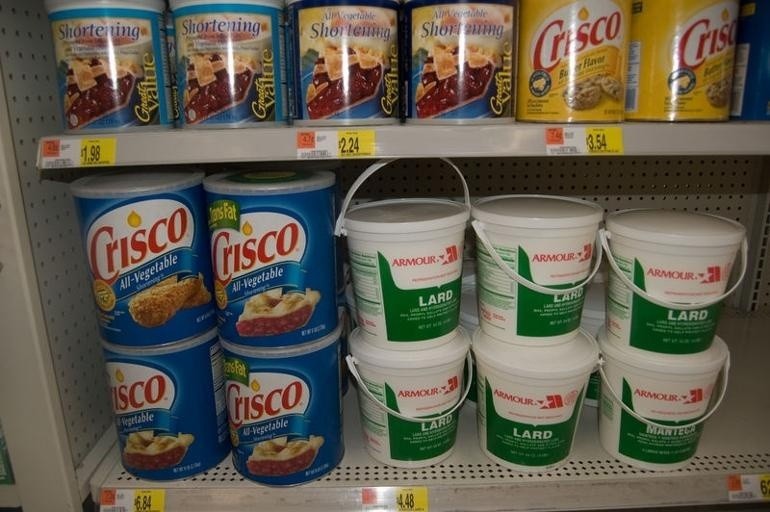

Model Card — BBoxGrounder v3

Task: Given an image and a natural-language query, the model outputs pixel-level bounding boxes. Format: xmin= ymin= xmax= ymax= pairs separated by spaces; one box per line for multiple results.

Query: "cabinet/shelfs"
xmin=0 ymin=0 xmax=769 ymax=512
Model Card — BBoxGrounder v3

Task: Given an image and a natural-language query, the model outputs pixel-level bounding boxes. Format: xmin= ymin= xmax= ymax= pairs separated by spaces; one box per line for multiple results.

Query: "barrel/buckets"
xmin=468 ymin=324 xmax=602 ymax=473
xmin=456 ymin=272 xmax=481 ymax=404
xmin=344 ymin=319 xmax=474 ymax=467
xmin=333 ymin=155 xmax=471 ymax=350
xmin=581 ymin=275 xmax=606 ymax=408
xmin=595 ymin=322 xmax=732 ymax=472
xmin=470 ymin=193 xmax=604 ymax=343
xmin=599 ymin=208 xmax=749 ymax=360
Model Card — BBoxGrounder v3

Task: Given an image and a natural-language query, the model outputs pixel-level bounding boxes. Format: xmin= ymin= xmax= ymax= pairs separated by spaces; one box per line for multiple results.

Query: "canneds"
xmin=170 ymin=0 xmax=288 ymax=129
xmin=70 ymin=170 xmax=345 ymax=488
xmin=400 ymin=1 xmax=515 ymax=126
xmin=626 ymin=0 xmax=740 ymax=122
xmin=287 ymin=0 xmax=400 ymax=126
xmin=47 ymin=6 xmax=172 ymax=134
xmin=516 ymin=0 xmax=625 ymax=122
xmin=730 ymin=1 xmax=769 ymax=122
